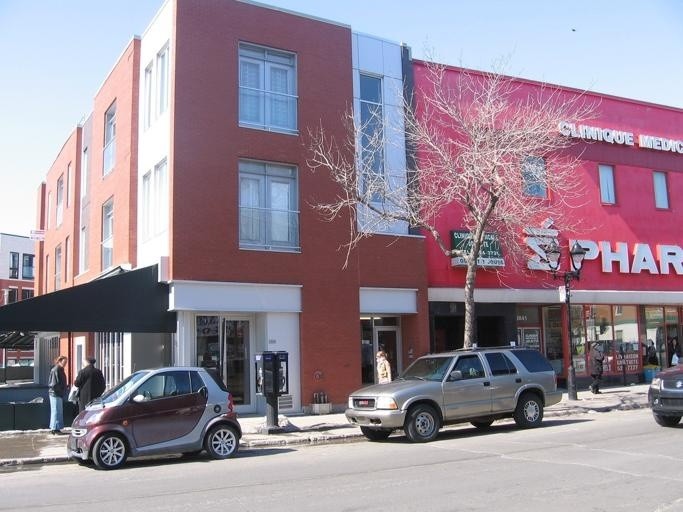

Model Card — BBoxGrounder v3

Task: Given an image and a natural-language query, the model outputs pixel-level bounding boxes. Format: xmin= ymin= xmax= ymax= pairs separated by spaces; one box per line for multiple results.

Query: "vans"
xmin=67 ymin=366 xmax=242 ymax=470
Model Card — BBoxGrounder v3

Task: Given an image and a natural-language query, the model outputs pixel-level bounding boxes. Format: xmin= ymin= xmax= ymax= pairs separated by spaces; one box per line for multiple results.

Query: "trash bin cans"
xmin=643 ymin=364 xmax=662 ymax=383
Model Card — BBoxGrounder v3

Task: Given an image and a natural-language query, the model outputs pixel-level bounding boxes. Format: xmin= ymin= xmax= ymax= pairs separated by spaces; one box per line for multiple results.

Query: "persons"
xmin=589 ymin=342 xmax=604 ymax=394
xmin=667 ymin=336 xmax=681 ymax=368
xmin=376 ymin=352 xmax=392 ymax=384
xmin=48 ymin=356 xmax=67 ymax=435
xmin=648 ymin=339 xmax=658 ymax=365
xmin=74 ymin=356 xmax=105 ymax=413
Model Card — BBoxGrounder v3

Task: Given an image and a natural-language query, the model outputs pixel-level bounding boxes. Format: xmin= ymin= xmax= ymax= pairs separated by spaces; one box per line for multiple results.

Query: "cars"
xmin=216 ymin=357 xmax=246 ymax=397
xmin=557 ymin=340 xmax=646 ymax=389
xmin=648 ymin=356 xmax=683 ymax=427
xmin=346 ymin=346 xmax=563 ymax=443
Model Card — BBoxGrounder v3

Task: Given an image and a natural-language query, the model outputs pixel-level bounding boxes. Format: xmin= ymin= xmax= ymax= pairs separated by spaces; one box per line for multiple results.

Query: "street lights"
xmin=545 ymin=238 xmax=586 ymax=401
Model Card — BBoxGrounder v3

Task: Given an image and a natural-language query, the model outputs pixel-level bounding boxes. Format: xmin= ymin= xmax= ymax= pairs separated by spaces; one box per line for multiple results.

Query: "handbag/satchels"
xmin=670 ymin=352 xmax=679 ymax=366
xmin=67 ymin=384 xmax=80 ymax=403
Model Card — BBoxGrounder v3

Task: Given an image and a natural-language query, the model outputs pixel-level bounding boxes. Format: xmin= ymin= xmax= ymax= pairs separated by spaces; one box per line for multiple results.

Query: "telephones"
xmin=278 ymin=367 xmax=285 ymax=389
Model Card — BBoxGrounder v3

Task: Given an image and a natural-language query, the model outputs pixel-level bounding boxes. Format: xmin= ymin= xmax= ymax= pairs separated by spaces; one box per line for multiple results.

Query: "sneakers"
xmin=588 ymin=384 xmax=601 ymax=394
xmin=51 ymin=430 xmax=65 ymax=435
